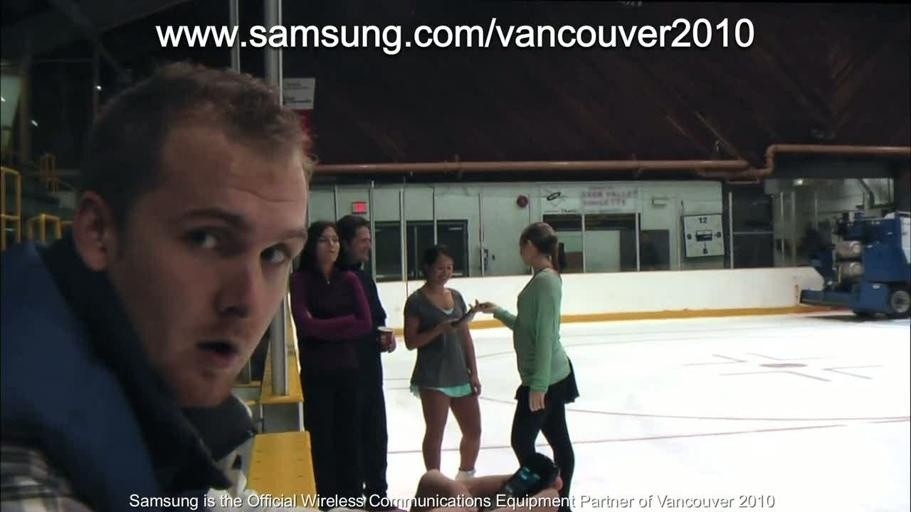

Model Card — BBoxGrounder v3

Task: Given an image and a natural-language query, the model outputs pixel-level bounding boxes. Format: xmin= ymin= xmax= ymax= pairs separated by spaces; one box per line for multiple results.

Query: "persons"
xmin=469 ymin=222 xmax=579 ymax=512
xmin=0 ymin=61 xmax=564 ymax=512
xmin=289 ymin=214 xmax=407 ymax=512
xmin=403 ymin=247 xmax=481 ymax=479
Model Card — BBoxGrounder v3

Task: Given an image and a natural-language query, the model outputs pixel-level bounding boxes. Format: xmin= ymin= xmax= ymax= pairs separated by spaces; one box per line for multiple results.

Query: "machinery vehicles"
xmin=799 ymin=210 xmax=910 ymax=319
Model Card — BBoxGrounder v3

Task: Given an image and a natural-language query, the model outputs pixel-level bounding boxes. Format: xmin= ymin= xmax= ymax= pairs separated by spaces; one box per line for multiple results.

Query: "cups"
xmin=378 ymin=324 xmax=393 ymax=350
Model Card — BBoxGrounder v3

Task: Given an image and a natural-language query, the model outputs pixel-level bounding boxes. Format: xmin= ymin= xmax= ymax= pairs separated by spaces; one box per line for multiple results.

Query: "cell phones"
xmin=477 ymin=453 xmax=560 ymax=511
